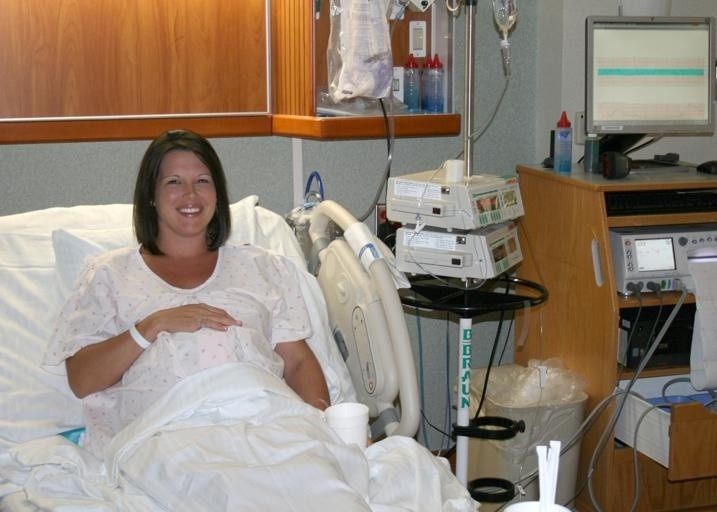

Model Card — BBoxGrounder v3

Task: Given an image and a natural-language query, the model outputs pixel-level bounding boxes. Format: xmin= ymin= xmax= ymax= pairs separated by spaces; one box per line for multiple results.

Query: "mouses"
xmin=697 ymin=160 xmax=716 ymax=174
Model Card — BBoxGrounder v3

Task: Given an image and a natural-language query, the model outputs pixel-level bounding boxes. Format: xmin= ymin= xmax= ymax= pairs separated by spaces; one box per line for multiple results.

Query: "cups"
xmin=324 ymin=401 xmax=370 ymax=452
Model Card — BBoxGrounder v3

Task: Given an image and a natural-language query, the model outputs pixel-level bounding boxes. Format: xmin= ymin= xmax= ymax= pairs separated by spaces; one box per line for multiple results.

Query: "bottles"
xmin=553 ymin=110 xmax=572 ymax=172
xmin=584 ymin=132 xmax=600 ymax=172
xmin=404 ymin=52 xmax=446 ymax=113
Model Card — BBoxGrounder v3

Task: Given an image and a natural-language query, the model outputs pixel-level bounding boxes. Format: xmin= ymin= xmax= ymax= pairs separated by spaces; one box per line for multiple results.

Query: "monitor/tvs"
xmin=583 ymin=16 xmax=716 ymax=136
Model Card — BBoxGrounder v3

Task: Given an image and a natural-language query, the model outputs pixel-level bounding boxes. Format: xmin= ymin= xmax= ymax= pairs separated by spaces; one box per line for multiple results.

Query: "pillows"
xmin=52 ymin=227 xmax=341 ymax=403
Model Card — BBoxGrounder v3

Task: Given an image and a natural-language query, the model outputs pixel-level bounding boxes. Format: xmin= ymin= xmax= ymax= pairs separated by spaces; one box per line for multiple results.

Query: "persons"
xmin=39 ymin=129 xmax=373 ymax=450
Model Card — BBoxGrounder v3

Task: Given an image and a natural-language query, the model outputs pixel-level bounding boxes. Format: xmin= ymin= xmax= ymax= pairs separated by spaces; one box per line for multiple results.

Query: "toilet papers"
xmin=446 ymin=160 xmax=463 ymax=180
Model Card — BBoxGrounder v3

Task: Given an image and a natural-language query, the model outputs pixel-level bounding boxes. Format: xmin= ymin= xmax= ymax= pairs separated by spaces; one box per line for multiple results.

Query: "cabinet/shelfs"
xmin=270 ymin=0 xmax=461 ymax=137
xmin=515 ymin=163 xmax=717 ymax=511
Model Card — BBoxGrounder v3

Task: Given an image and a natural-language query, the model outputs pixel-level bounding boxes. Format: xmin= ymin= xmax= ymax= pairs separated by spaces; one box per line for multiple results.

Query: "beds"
xmin=0 ymin=197 xmax=481 ymax=510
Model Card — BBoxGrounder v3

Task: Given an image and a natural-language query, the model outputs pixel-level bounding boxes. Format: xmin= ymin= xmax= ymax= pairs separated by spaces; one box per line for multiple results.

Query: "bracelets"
xmin=130 ymin=319 xmax=153 ymax=353
